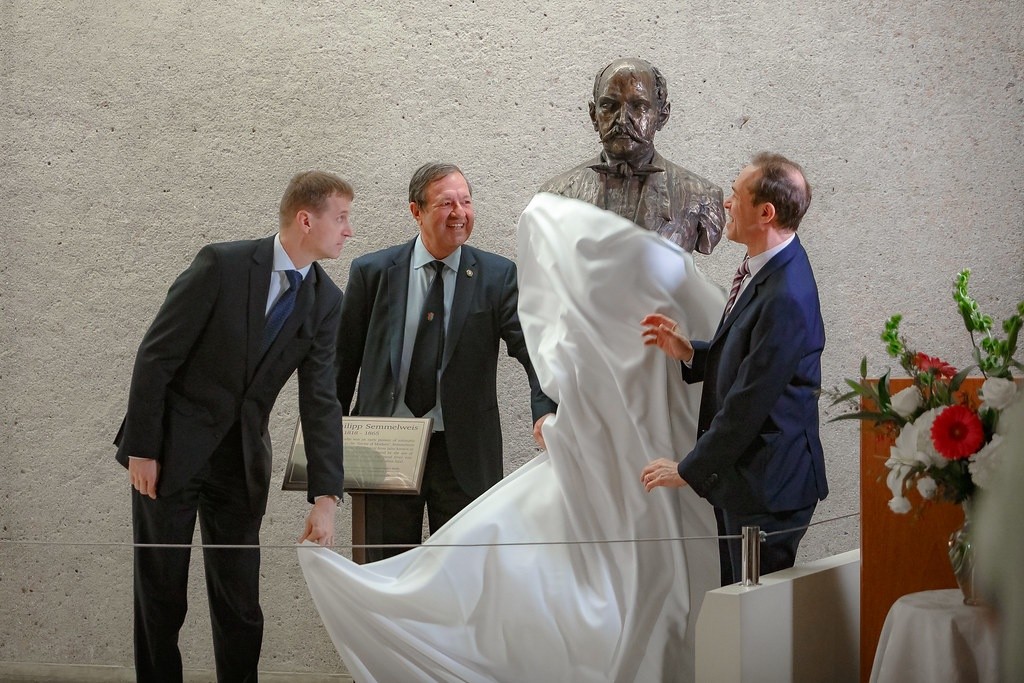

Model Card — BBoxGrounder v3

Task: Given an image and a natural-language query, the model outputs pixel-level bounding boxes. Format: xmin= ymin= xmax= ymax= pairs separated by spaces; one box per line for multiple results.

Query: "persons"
xmin=113 ymin=170 xmax=354 ymax=683
xmin=539 ymin=56 xmax=726 ymax=256
xmin=332 ymin=162 xmax=558 ymax=560
xmin=639 ymin=150 xmax=830 ymax=587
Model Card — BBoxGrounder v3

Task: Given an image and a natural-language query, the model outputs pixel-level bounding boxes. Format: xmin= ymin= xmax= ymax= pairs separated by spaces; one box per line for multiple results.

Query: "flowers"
xmin=813 ymin=270 xmax=1024 ymax=518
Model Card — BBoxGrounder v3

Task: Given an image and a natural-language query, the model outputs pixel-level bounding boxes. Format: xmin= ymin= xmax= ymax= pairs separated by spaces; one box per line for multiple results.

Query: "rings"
xmin=647 ymin=474 xmax=650 ymax=482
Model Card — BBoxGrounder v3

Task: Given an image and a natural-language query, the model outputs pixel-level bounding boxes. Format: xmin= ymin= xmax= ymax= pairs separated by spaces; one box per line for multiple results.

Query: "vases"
xmin=946 ymin=496 xmax=990 ymax=606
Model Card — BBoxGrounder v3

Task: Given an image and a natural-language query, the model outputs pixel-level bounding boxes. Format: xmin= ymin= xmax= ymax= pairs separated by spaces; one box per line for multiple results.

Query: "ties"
xmin=404 ymin=260 xmax=446 ymax=418
xmin=256 ymin=270 xmax=303 ymax=363
xmin=723 ymin=257 xmax=751 ymax=324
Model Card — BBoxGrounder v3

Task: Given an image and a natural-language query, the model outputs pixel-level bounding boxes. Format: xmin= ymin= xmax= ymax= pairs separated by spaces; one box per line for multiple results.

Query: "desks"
xmin=869 ymin=588 xmax=1024 ymax=683
